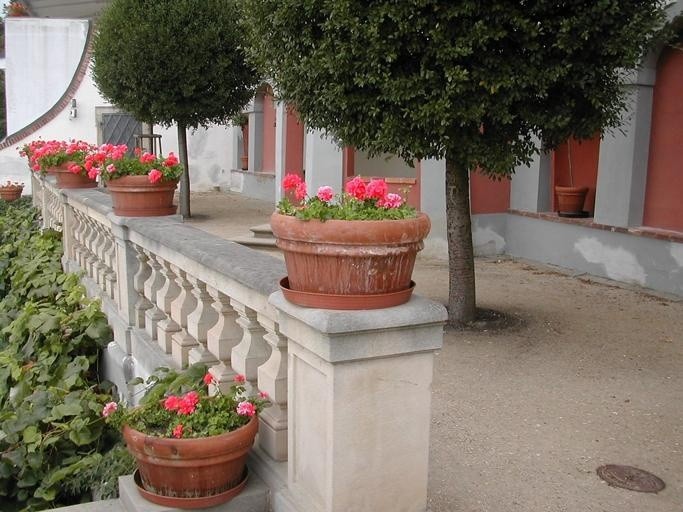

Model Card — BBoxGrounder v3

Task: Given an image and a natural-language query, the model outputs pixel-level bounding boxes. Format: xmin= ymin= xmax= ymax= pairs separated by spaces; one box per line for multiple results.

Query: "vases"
xmin=0 ymin=189 xmax=23 ymax=201
xmin=29 ymin=157 xmax=36 ymax=166
xmin=270 ymin=212 xmax=432 ymax=294
xmin=123 ymin=415 xmax=258 ymax=498
xmin=554 ymin=186 xmax=588 ymax=212
xmin=46 ymin=164 xmax=69 ymax=174
xmin=8 ymin=12 xmax=22 ymax=16
xmin=106 ymin=175 xmax=176 ymax=208
xmin=53 ymin=169 xmax=96 ymax=184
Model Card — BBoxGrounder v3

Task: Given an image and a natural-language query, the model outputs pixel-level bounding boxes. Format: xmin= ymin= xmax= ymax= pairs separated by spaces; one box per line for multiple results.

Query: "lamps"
xmin=70 ymin=98 xmax=77 ymax=117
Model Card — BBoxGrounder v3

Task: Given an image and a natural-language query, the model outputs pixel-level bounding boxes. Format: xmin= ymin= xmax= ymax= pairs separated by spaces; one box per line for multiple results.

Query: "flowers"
xmin=102 ymin=362 xmax=272 ymax=438
xmin=1 ymin=0 xmax=27 ymax=14
xmin=16 ymin=136 xmax=46 ymax=158
xmin=88 ymin=144 xmax=183 ymax=185
xmin=55 ymin=139 xmax=98 ymax=174
xmin=0 ymin=180 xmax=25 ymax=189
xmin=276 ymin=173 xmax=418 ymax=221
xmin=31 ymin=140 xmax=68 ymax=181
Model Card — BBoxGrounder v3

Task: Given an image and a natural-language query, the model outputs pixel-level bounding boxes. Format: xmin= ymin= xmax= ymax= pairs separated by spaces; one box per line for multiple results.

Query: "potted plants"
xmin=231 ymin=113 xmax=248 ymax=169
